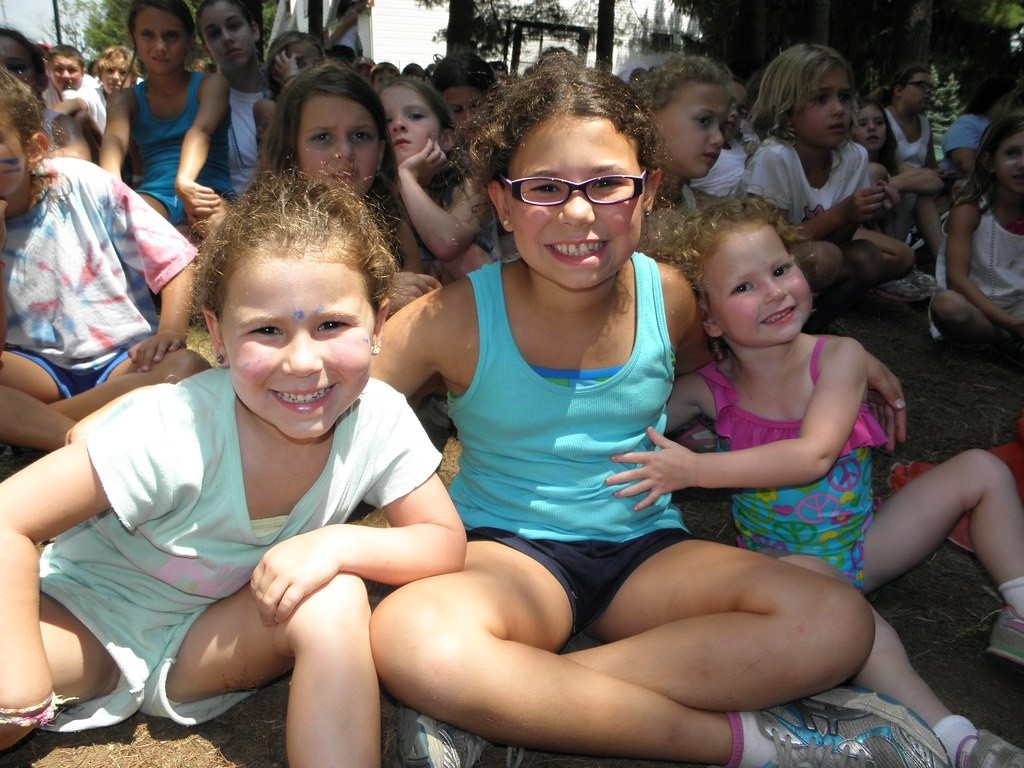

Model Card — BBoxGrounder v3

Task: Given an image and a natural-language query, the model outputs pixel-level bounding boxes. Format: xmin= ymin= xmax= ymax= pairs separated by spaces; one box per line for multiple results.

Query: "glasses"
xmin=906 ymin=80 xmax=932 ymax=89
xmin=499 ymin=168 xmax=648 ymax=206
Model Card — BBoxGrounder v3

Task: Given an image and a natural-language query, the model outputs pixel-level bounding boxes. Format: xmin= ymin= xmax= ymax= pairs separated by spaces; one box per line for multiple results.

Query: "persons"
xmin=628 ymin=44 xmax=1024 ymax=367
xmin=606 ymin=201 xmax=1024 ymax=768
xmin=0 ymin=171 xmax=466 ymax=768
xmin=369 ymin=48 xmax=951 ymax=768
xmin=0 ymin=0 xmax=574 ymax=452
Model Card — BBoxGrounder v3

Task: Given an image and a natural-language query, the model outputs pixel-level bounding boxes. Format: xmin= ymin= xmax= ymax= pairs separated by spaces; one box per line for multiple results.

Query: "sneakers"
xmin=749 ymin=686 xmax=951 ymax=768
xmin=399 ymin=702 xmax=487 ymax=768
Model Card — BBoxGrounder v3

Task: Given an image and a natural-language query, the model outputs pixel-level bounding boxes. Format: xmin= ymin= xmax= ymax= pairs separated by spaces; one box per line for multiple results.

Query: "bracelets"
xmin=0 ymin=689 xmax=80 ymax=727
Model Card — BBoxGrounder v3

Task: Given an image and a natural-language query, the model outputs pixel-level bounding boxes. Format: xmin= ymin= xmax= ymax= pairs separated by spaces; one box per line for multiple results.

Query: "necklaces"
xmin=228 ymin=71 xmax=268 ymax=175
xmin=990 ymin=202 xmax=996 ymax=299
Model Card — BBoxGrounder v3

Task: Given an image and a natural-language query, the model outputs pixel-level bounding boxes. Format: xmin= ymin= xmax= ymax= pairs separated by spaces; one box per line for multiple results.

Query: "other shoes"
xmin=908 ymin=231 xmax=923 ymax=251
xmin=883 ymin=274 xmax=937 ymax=301
xmin=954 ymin=732 xmax=1024 ymax=768
xmin=986 ymin=608 xmax=1024 ymax=667
